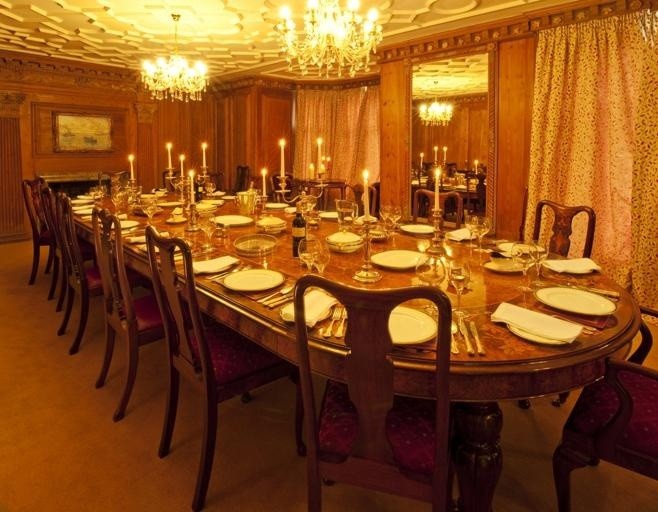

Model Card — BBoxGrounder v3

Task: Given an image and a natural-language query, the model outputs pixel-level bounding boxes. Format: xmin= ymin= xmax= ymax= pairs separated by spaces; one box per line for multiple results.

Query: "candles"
xmin=420 ymin=152 xmax=424 ymax=168
xmin=202 ymin=143 xmax=207 ymax=167
xmin=128 ymin=154 xmax=135 ymax=180
xmin=279 ymin=139 xmax=286 ymax=178
xmin=180 ymin=155 xmax=185 ymax=176
xmin=166 ymin=143 xmax=172 ymax=168
xmin=317 ymin=139 xmax=322 ymax=173
xmin=434 ymin=146 xmax=438 ymax=161
xmin=443 ymin=146 xmax=448 ymax=160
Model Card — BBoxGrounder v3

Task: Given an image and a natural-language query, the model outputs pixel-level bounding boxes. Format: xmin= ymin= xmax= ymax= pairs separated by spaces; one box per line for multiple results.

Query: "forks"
xmin=334 ymin=307 xmax=347 ymax=337
xmin=323 ymin=307 xmax=342 ymax=337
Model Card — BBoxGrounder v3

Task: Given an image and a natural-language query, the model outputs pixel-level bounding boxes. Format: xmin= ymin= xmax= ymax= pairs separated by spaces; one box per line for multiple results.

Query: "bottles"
xmin=291 ymin=201 xmax=307 ymax=258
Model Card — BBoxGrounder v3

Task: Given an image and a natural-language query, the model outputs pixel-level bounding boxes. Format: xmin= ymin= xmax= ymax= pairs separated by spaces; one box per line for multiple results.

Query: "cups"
xmin=311 ymin=242 xmax=331 ymax=276
xmin=414 ymin=256 xmax=446 ymax=287
xmin=298 ymin=238 xmax=320 ymax=272
xmin=336 ymin=199 xmax=359 ymax=229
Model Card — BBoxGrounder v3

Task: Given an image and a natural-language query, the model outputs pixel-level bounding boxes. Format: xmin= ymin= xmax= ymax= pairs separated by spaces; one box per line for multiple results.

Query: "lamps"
xmin=417 ymin=80 xmax=454 ymax=127
xmin=141 ymin=13 xmax=210 ymax=103
xmin=272 ymin=0 xmax=384 ymax=78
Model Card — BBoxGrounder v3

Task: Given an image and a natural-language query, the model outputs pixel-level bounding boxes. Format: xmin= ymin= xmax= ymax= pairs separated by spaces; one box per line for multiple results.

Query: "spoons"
xmin=256 ymin=285 xmax=294 ymax=304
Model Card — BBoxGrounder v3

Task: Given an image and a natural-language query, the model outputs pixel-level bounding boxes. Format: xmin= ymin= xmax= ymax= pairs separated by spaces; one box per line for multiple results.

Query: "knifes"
xmin=456 ymin=317 xmax=475 ymax=353
xmin=469 ymin=320 xmax=485 ymax=354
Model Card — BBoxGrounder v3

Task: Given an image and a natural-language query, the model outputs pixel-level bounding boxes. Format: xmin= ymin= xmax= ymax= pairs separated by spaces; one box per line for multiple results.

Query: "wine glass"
xmin=447 ymin=259 xmax=472 ymax=318
xmin=465 ymin=215 xmax=479 ymax=256
xmin=528 ymin=240 xmax=549 ymax=287
xmin=475 ymin=217 xmax=492 ymax=260
xmin=511 ymin=241 xmax=536 ymax=293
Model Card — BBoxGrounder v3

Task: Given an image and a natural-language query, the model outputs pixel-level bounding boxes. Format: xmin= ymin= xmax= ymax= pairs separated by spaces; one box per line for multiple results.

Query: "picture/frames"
xmin=52 ymin=110 xmax=115 ymax=154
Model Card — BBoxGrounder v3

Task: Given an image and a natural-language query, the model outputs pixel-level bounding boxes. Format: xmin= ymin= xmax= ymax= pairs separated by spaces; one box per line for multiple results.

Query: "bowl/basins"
xmin=325 ymin=230 xmax=366 ymax=254
xmin=255 ymin=214 xmax=287 ymax=235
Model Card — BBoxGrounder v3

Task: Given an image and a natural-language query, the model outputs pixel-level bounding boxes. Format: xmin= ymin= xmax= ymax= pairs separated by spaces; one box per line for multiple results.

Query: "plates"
xmin=100 ymin=220 xmax=139 ymax=230
xmin=497 ymin=243 xmax=546 ymax=253
xmin=400 ymin=224 xmax=435 ymax=235
xmin=541 ymin=259 xmax=593 ymax=275
xmin=279 ymin=302 xmax=332 ymax=324
xmin=388 ymin=306 xmax=439 ymax=346
xmin=452 ymin=227 xmax=490 ymax=240
xmin=166 ymin=218 xmax=188 ymax=223
xmin=320 ymin=212 xmax=340 ymax=218
xmin=484 ymin=260 xmax=530 ymax=274
xmin=223 ymin=268 xmax=285 ymax=292
xmin=67 ymin=184 xmax=264 ymax=221
xmin=533 ymin=287 xmax=617 ymax=317
xmin=210 ymin=214 xmax=254 ymax=227
xmin=370 ymin=249 xmax=431 ymax=269
xmin=411 ymin=175 xmax=479 ymax=191
xmin=233 ymin=234 xmax=279 ymax=257
xmin=191 ymin=258 xmax=232 ymax=274
xmin=506 ymin=323 xmax=566 ymax=346
xmin=267 ymin=203 xmax=289 ymax=209
xmin=142 ymin=240 xmax=194 ymax=253
